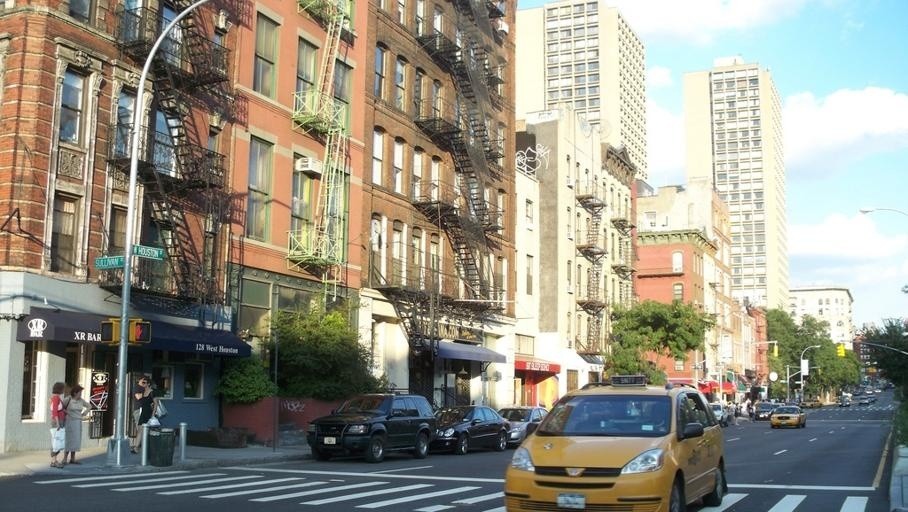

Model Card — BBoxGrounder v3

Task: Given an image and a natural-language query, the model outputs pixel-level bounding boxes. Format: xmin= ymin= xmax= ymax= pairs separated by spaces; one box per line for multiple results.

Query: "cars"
xmin=838 ymin=383 xmax=895 ymax=407
xmin=429 ymin=403 xmax=512 ymax=456
xmin=502 ymin=372 xmax=729 ymax=511
xmin=706 ymin=398 xmax=823 ymax=430
xmin=496 ymin=405 xmax=551 ymax=448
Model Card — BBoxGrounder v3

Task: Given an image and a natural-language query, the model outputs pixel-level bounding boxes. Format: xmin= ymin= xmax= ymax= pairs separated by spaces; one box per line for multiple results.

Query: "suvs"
xmin=301 ymin=391 xmax=440 ymax=466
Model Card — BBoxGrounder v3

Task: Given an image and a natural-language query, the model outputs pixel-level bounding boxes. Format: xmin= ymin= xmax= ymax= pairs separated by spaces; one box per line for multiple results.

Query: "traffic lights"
xmin=836 ymin=344 xmax=846 ymax=358
xmin=128 ymin=319 xmax=153 ymax=345
xmin=99 ymin=318 xmax=121 ymax=345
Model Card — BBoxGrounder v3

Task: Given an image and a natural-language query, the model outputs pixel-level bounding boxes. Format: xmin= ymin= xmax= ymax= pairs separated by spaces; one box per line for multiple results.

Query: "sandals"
xmin=131 ymin=447 xmax=139 ymax=453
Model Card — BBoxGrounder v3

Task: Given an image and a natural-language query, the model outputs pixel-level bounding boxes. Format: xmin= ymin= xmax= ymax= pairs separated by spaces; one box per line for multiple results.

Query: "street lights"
xmin=800 ymin=344 xmax=821 ymax=401
xmin=859 ymin=206 xmax=907 ymax=220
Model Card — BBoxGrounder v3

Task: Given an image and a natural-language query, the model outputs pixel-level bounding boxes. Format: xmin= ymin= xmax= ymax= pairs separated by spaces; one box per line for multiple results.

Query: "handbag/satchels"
xmin=50 ymin=401 xmax=63 ymax=411
xmin=150 ymin=399 xmax=168 ymax=419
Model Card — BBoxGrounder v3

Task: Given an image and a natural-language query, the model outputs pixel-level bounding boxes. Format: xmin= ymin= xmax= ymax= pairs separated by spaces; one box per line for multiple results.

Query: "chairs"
xmin=651 ymin=402 xmax=669 ymax=429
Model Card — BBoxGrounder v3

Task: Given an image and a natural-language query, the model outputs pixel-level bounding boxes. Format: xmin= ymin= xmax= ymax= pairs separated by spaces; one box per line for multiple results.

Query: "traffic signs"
xmin=94 ymin=256 xmax=127 ymax=271
xmin=131 ymin=245 xmax=166 ymax=261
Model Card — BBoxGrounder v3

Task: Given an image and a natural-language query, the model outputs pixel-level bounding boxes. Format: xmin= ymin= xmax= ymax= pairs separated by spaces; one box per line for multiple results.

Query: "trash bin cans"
xmin=149 ymin=426 xmax=175 ymax=467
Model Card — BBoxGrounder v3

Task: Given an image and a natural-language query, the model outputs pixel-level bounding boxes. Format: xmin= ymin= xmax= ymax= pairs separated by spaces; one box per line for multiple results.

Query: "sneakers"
xmin=50 ymin=460 xmax=81 ymax=468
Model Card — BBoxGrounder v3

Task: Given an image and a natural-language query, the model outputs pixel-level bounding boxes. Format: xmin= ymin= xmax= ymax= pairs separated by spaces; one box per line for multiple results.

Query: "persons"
xmin=49 ymin=383 xmax=65 ymax=467
xmin=724 ymin=398 xmax=779 ymax=426
xmin=130 ymin=376 xmax=157 ymax=452
xmin=62 ymin=385 xmax=92 ymax=464
